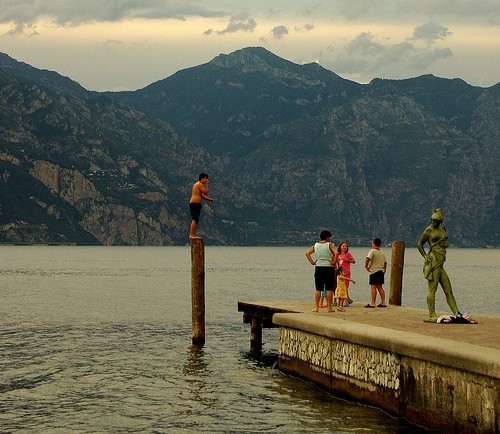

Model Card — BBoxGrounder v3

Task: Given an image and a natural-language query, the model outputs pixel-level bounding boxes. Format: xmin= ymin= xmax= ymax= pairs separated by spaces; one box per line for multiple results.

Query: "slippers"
xmin=364 ymin=304 xmax=375 ymax=308
xmin=377 ymin=304 xmax=387 ymax=307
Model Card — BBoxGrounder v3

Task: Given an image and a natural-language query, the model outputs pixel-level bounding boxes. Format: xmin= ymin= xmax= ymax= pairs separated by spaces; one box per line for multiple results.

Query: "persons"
xmin=306 ymin=230 xmax=338 ymax=312
xmin=418 ymin=208 xmax=465 ymax=323
xmin=365 ymin=238 xmax=387 ymax=308
xmin=336 ymin=266 xmax=355 ymax=312
xmin=335 ymin=242 xmax=356 ymax=307
xmin=188 ymin=173 xmax=214 ymax=239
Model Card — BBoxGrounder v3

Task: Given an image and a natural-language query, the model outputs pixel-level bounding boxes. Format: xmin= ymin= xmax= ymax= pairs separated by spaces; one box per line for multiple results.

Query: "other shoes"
xmin=312 ymin=308 xmax=318 ymax=312
xmin=328 ymin=309 xmax=335 ymax=312
